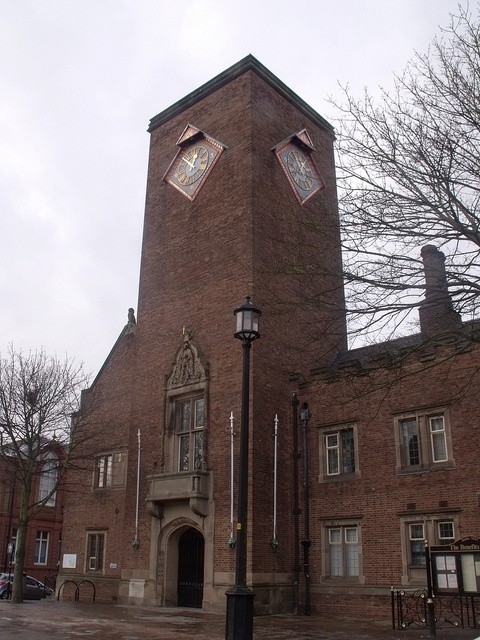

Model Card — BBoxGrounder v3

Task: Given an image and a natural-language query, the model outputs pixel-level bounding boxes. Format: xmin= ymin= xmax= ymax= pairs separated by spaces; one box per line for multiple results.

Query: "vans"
xmin=0 ymin=573 xmax=53 ymax=600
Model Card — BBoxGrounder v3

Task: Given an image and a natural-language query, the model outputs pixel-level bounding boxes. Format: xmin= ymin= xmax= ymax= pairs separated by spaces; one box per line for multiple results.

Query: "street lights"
xmin=7 ymin=543 xmax=15 ymax=599
xmin=300 ymin=408 xmax=311 ymax=616
xmin=225 ymin=296 xmax=261 ymax=640
